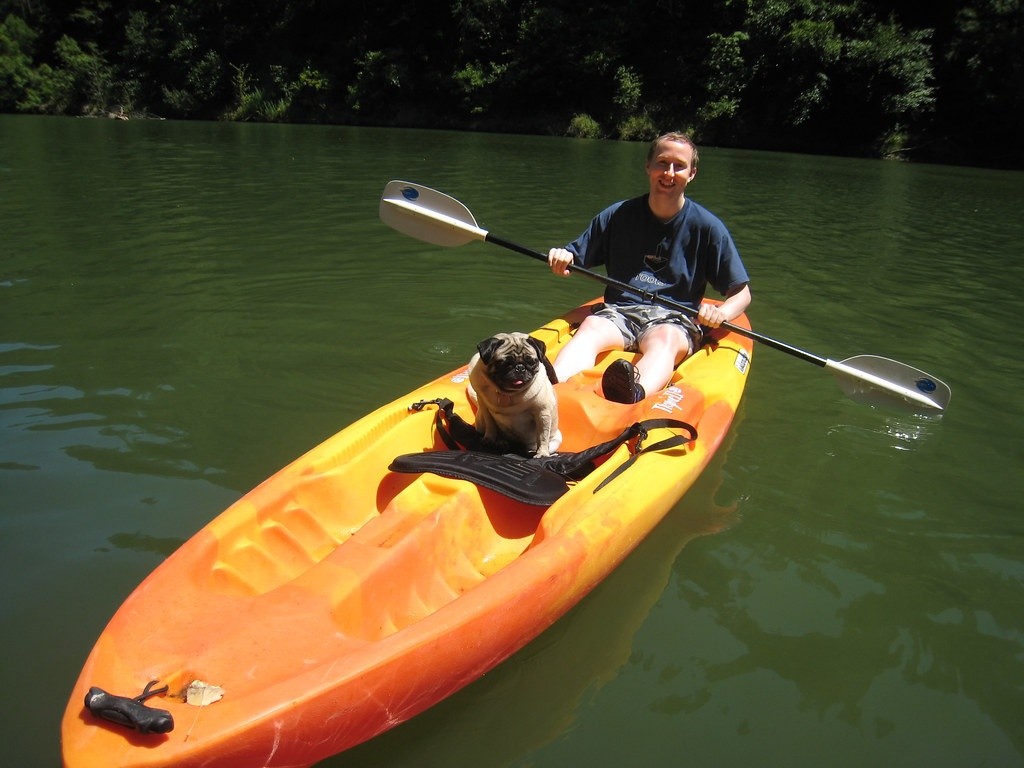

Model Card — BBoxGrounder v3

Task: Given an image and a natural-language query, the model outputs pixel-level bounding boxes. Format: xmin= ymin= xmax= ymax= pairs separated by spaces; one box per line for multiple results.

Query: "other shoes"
xmin=526 ymin=337 xmax=558 ymax=385
xmin=602 ymin=358 xmax=645 ymax=404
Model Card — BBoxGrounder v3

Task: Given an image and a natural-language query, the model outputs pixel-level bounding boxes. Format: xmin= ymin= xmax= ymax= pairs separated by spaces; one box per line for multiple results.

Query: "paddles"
xmin=378 ymin=179 xmax=952 ymax=420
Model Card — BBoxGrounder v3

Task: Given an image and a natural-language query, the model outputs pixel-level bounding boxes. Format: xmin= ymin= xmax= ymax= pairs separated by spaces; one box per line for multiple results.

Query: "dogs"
xmin=467 ymin=332 xmax=562 ymax=458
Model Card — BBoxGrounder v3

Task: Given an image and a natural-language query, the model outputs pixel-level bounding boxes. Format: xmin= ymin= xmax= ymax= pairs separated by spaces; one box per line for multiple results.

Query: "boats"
xmin=60 ymin=282 xmax=754 ymax=768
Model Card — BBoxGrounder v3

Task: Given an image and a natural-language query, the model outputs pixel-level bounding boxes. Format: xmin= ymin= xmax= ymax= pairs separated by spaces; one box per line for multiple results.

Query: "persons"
xmin=546 ymin=134 xmax=751 ymax=404
